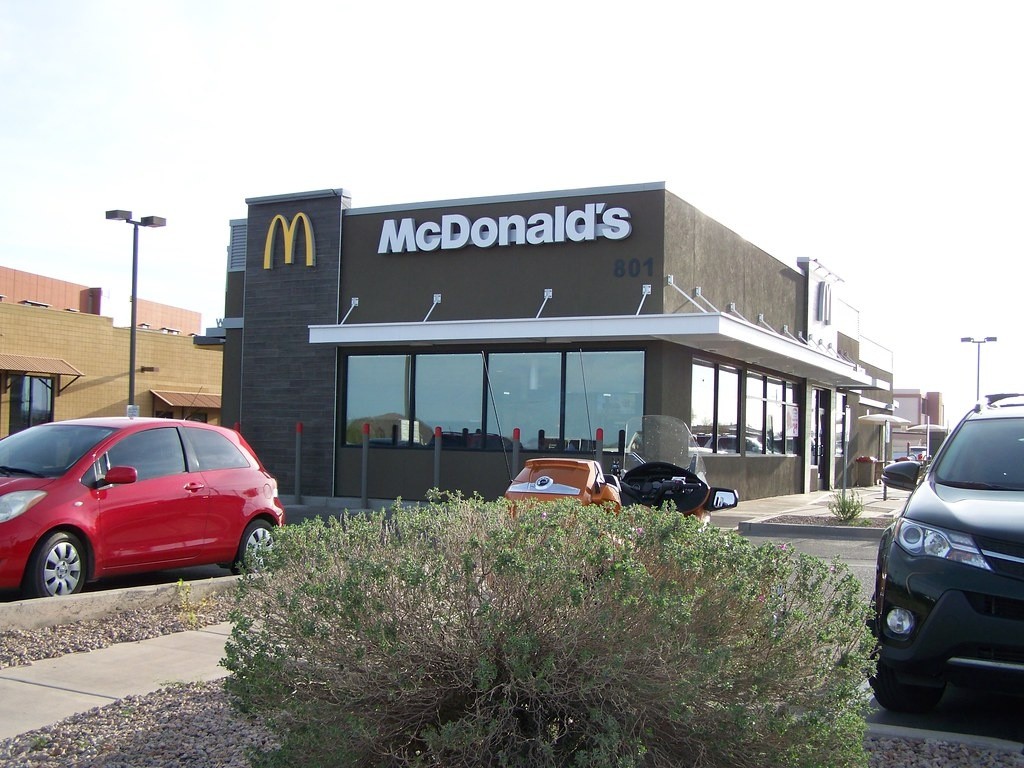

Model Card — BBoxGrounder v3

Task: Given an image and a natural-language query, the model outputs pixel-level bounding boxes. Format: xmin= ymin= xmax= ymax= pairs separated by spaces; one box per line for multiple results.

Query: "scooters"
xmin=505 ymin=415 xmax=738 ymax=528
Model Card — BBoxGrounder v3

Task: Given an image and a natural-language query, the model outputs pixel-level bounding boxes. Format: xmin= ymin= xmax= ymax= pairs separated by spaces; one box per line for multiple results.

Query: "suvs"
xmin=857 ymin=394 xmax=1024 ymax=716
xmin=691 ymin=433 xmax=773 ymax=454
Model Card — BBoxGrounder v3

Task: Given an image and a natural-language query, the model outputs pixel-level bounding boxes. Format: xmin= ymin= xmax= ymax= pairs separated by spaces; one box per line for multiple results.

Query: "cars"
xmin=354 ymin=432 xmax=568 ymax=450
xmin=0 ymin=417 xmax=286 ymax=599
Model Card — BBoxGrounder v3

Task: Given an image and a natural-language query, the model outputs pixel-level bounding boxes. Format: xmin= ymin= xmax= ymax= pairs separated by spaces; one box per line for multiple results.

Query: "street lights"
xmin=106 ymin=211 xmax=165 ymax=417
xmin=961 ymin=337 xmax=997 ymax=403
